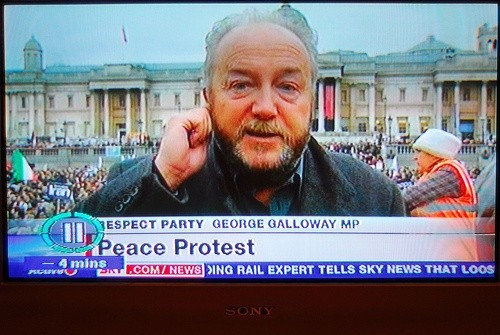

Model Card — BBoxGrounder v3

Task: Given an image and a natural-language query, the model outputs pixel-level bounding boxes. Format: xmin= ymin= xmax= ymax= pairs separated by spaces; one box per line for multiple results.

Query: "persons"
xmin=4 ymin=5 xmax=494 ymax=259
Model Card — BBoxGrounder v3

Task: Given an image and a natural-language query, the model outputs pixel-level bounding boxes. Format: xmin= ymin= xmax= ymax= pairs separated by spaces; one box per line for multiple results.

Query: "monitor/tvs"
xmin=1 ymin=0 xmax=500 ymax=334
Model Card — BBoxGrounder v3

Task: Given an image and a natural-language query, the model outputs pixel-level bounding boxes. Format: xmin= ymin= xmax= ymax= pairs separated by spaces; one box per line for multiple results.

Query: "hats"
xmin=412 ymin=127 xmax=463 ymax=160
